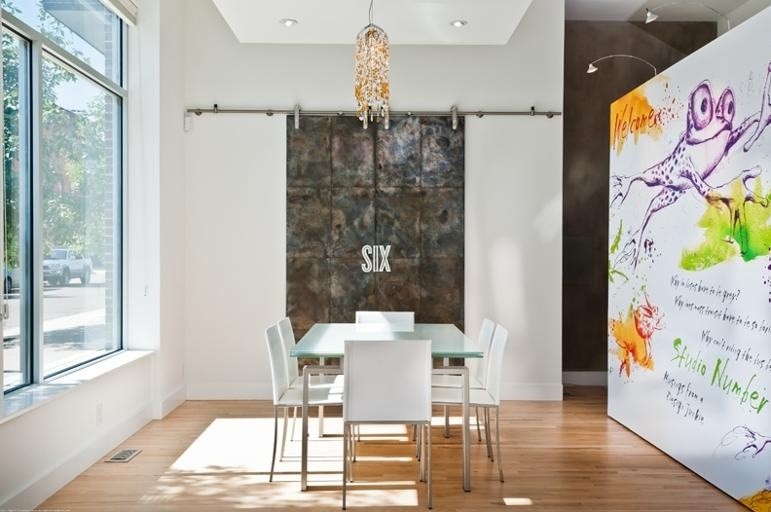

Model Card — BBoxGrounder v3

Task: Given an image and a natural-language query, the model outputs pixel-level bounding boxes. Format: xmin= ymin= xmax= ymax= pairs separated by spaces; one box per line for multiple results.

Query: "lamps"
xmin=353 ymin=0 xmax=395 ymax=133
xmin=643 ymin=2 xmax=731 ymax=32
xmin=586 ymin=54 xmax=656 ymax=81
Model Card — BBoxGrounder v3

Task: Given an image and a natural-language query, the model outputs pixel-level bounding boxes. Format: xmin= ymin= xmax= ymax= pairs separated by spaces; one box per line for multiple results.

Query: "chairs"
xmin=430 ymin=317 xmax=506 ymax=478
xmin=340 ymin=338 xmax=436 ymax=512
xmin=354 ymin=310 xmax=416 ymax=326
xmin=261 ymin=312 xmax=344 ymax=483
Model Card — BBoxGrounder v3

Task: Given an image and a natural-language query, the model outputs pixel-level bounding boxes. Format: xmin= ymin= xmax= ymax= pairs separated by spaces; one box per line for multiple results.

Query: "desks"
xmin=290 ymin=321 xmax=485 ymax=494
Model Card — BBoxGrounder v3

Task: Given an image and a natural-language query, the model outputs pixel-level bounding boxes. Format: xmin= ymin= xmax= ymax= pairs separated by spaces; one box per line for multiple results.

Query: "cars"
xmin=3 ymin=264 xmax=21 ymax=292
xmin=42 ymin=248 xmax=92 ymax=285
